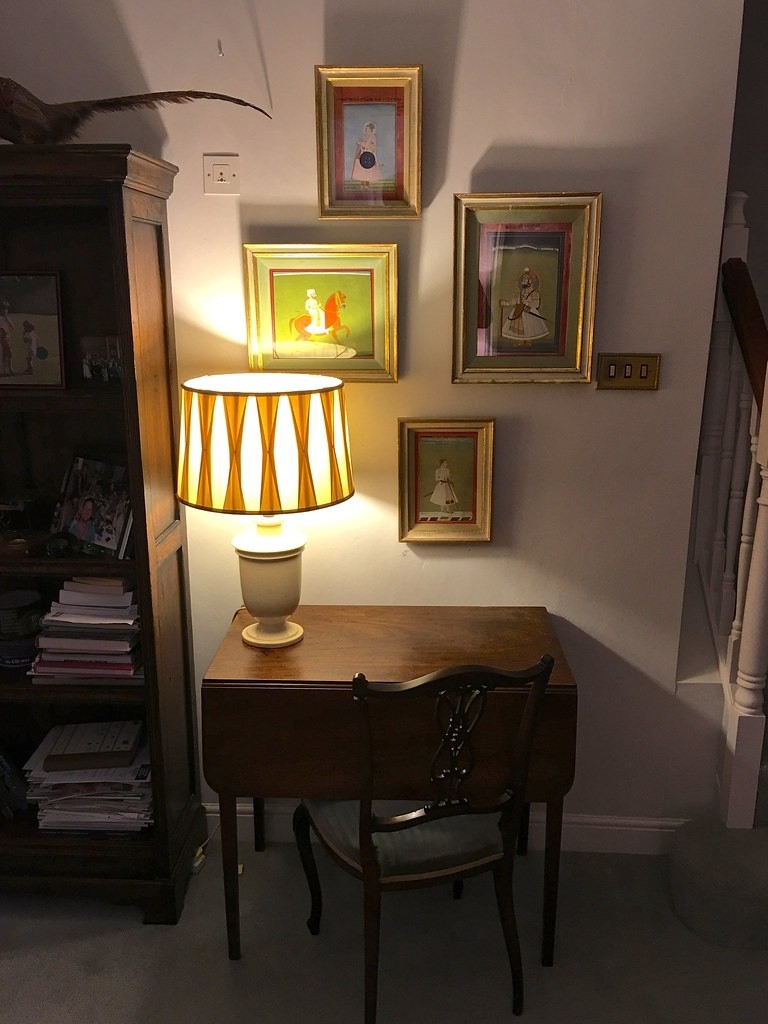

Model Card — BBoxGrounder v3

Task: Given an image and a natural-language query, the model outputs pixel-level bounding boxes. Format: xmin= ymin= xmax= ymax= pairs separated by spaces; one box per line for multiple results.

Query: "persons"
xmin=80 ymin=351 xmax=121 ymax=384
xmin=22 ymin=319 xmax=39 ymax=375
xmin=60 ymin=479 xmax=128 ymax=542
xmin=0 ymin=300 xmax=16 ymax=377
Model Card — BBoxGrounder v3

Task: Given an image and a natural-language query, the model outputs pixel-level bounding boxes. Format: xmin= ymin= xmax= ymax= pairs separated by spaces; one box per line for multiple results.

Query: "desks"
xmin=200 ymin=602 xmax=580 ymax=967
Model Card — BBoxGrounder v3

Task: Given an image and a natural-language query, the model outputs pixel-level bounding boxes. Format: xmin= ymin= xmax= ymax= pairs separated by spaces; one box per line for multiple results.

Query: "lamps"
xmin=176 ymin=370 xmax=355 ymax=649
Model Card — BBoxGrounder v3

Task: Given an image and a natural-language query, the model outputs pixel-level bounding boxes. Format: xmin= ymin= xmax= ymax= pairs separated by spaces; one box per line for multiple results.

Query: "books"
xmin=25 ymin=575 xmax=145 ymax=686
xmin=23 ymin=720 xmax=154 ymax=831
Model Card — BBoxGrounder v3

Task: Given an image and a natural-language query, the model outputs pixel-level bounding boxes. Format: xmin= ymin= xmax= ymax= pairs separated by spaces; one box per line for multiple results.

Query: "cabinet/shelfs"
xmin=0 ymin=142 xmax=209 ymax=924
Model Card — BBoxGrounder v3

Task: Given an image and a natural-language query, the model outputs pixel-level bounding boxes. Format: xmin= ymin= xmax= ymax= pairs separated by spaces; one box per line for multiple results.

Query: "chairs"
xmin=291 ymin=655 xmax=555 ymax=1024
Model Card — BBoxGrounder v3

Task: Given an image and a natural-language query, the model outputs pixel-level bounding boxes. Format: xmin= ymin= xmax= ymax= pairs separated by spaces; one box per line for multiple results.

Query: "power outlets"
xmin=203 ymin=156 xmax=242 ymax=195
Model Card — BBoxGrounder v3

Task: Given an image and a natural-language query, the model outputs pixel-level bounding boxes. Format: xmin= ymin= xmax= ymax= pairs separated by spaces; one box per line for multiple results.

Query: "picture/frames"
xmin=242 ymin=243 xmax=399 ymax=384
xmin=315 ymin=65 xmax=422 ymax=220
xmin=396 ymin=414 xmax=494 ymax=544
xmin=0 ymin=270 xmax=67 ymax=391
xmin=450 ymin=190 xmax=602 ymax=385
xmin=49 ymin=445 xmax=132 ymax=560
